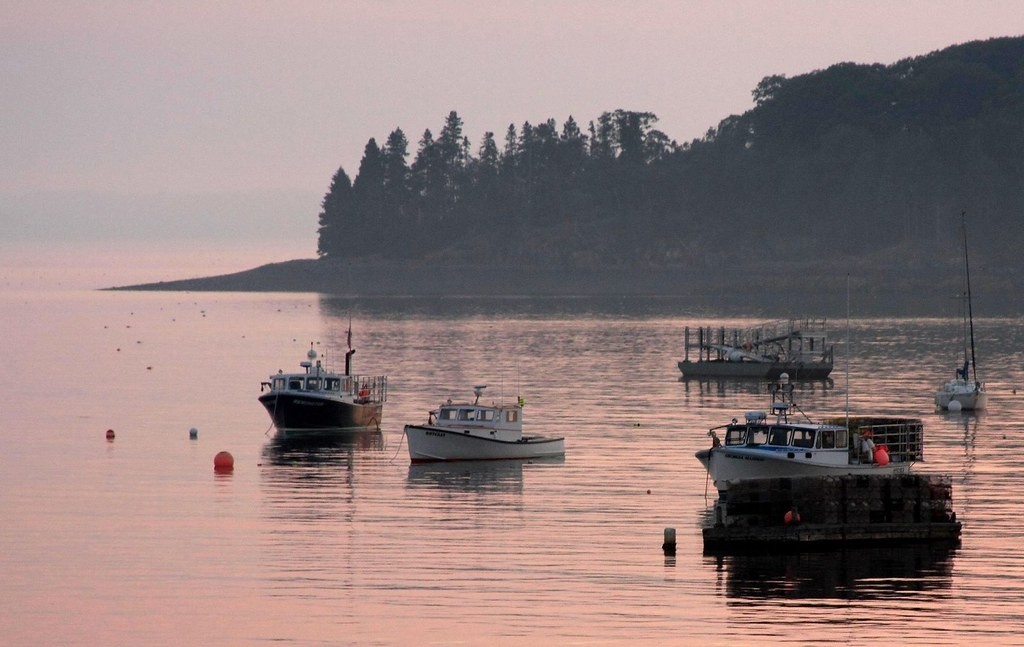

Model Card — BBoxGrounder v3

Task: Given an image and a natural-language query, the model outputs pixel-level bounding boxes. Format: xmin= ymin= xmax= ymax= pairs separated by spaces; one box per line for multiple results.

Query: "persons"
xmin=857 ymin=431 xmax=877 ymax=465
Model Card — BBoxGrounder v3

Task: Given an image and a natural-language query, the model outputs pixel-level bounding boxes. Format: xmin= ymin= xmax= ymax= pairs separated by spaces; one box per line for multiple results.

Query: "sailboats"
xmin=936 ymin=211 xmax=995 ymax=413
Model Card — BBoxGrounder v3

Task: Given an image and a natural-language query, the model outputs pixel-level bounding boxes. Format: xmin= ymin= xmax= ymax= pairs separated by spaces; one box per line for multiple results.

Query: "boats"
xmin=405 ymin=386 xmax=568 ymax=466
xmin=697 ymin=472 xmax=966 ymax=558
xmin=256 ymin=308 xmax=389 ymax=441
xmin=693 ymin=373 xmax=912 ymax=493
xmin=675 ymin=321 xmax=837 ymax=378
